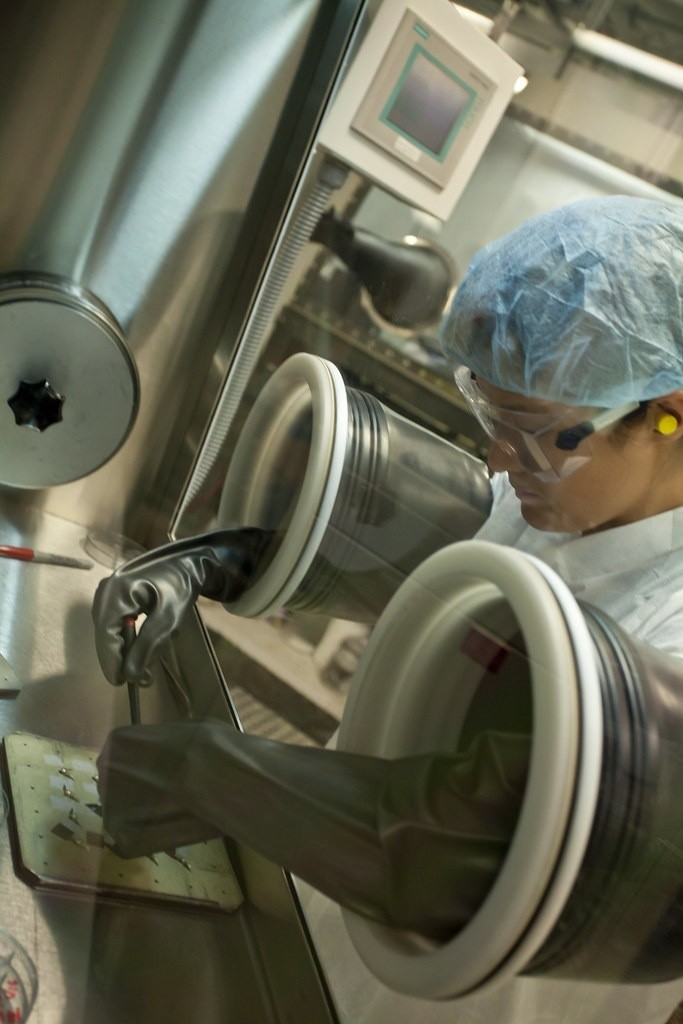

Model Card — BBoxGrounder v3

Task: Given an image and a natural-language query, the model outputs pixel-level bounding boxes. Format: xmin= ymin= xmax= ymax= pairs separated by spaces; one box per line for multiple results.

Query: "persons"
xmin=89 ymin=195 xmax=683 ymax=1024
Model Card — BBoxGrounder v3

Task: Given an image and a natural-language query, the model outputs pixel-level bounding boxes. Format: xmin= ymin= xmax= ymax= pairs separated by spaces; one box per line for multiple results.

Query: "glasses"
xmin=448 ymin=364 xmax=659 ymax=486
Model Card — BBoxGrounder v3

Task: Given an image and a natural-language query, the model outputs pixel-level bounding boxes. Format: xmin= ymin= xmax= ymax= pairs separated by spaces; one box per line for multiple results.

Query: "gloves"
xmin=93 ymin=718 xmax=521 ymax=939
xmin=91 ymin=522 xmax=271 ymax=691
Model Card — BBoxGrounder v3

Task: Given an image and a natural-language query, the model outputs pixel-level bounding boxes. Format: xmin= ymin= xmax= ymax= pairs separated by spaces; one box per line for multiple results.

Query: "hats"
xmin=437 ymin=196 xmax=683 ymax=409
